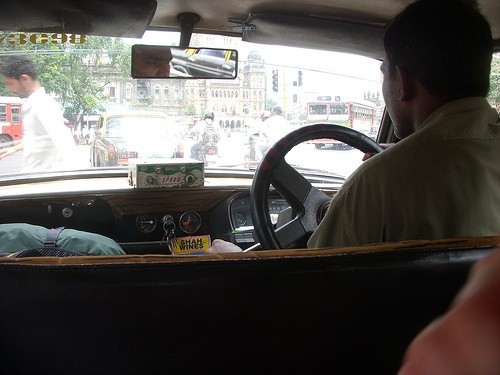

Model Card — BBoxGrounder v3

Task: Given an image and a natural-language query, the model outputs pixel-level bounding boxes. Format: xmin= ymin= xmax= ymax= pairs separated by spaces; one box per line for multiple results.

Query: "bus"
xmin=307 ymin=99 xmax=378 ymax=150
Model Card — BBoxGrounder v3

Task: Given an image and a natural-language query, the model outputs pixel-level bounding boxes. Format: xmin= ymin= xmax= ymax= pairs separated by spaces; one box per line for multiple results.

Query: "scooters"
xmin=187 ymin=132 xmax=219 ymax=170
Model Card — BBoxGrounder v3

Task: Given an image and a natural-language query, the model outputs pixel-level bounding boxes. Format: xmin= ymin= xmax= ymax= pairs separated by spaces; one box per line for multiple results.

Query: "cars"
xmin=91 ymin=111 xmax=186 ymax=167
xmin=0 ymin=100 xmax=24 ymax=150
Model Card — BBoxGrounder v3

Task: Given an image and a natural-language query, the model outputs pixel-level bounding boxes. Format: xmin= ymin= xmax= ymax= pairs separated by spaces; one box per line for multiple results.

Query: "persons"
xmin=205 ymin=0 xmax=500 ymax=254
xmin=133 ymin=46 xmax=173 ymax=77
xmin=189 ymin=111 xmax=223 ymax=159
xmin=251 ymin=105 xmax=295 ymax=162
xmin=0 ymin=55 xmax=78 ymax=174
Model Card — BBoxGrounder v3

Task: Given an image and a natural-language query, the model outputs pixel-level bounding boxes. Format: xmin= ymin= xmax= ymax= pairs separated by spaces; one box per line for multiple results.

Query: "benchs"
xmin=0 ymin=236 xmax=500 ymax=375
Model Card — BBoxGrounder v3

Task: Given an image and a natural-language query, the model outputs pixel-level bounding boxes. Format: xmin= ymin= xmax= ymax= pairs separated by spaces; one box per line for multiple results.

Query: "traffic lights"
xmin=272 ymin=69 xmax=279 ymax=92
